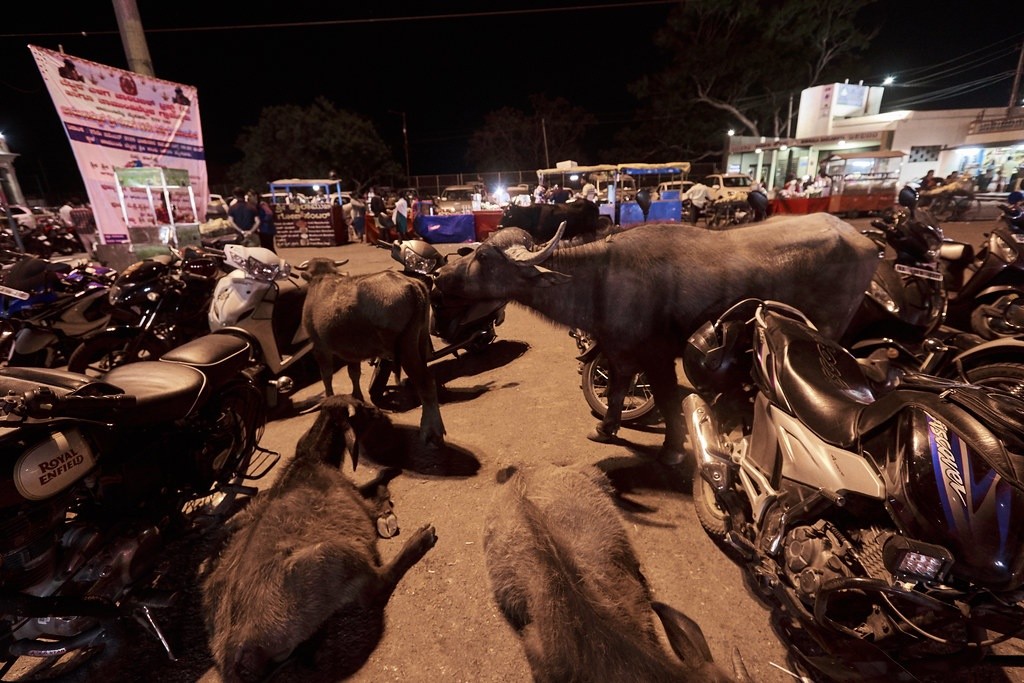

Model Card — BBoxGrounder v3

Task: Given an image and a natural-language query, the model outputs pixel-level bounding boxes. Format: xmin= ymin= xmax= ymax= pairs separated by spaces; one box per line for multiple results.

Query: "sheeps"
xmin=483 ymin=463 xmax=750 ymax=683
xmin=199 ymin=395 xmax=436 ymax=683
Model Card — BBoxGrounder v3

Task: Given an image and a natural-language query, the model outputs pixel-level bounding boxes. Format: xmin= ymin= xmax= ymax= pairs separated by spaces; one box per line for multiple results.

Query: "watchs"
xmin=241 ymin=230 xmax=244 ymax=234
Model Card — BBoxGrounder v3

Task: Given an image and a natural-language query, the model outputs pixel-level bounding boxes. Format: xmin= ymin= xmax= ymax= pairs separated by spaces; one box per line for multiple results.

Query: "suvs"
xmin=699 ymin=173 xmax=754 ymax=218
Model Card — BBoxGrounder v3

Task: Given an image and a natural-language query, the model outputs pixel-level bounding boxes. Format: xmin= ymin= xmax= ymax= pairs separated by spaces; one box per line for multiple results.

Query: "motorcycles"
xmin=569 ymin=324 xmax=673 ymax=422
xmin=1 ymin=327 xmax=294 ymax=679
xmin=369 ymin=212 xmax=506 ymax=398
xmin=1 ymin=231 xmax=342 ymax=383
xmin=704 ymin=195 xmax=753 ymax=229
xmin=840 ymin=184 xmax=1024 ymax=402
xmin=0 ymin=217 xmax=76 ymax=260
xmin=680 ymin=300 xmax=1024 ymax=683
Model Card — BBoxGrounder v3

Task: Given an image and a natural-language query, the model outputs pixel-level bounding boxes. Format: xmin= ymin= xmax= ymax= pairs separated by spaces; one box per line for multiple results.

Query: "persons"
xmin=59 ymin=199 xmax=98 ymax=260
xmin=685 ymin=178 xmax=714 ymax=227
xmin=228 ymin=186 xmax=278 ymax=255
xmin=920 ymin=157 xmax=1024 ymax=236
xmin=747 ymin=168 xmax=832 ymax=220
xmin=470 ymin=185 xmax=574 ymax=213
xmin=285 ymin=187 xmax=424 ymax=247
xmin=575 ymin=176 xmax=596 ymax=203
xmin=619 ymin=167 xmax=637 ymax=202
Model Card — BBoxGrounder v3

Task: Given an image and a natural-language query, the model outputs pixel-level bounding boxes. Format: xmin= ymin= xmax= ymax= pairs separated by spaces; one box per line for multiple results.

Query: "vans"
xmin=588 ymin=173 xmax=636 ymax=200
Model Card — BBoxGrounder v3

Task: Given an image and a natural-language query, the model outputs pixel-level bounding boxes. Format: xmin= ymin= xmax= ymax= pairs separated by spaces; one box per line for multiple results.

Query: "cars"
xmin=651 ymin=181 xmax=696 ymax=222
xmin=437 ymin=185 xmax=575 ymax=214
xmin=208 ymin=190 xmax=366 ymax=225
xmin=1 ymin=204 xmax=59 ymax=237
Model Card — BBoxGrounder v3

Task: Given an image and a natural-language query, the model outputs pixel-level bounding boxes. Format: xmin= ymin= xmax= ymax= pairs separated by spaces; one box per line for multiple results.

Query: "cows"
xmin=294 ymin=258 xmax=447 ymax=450
xmin=433 ymin=197 xmax=879 ymax=458
